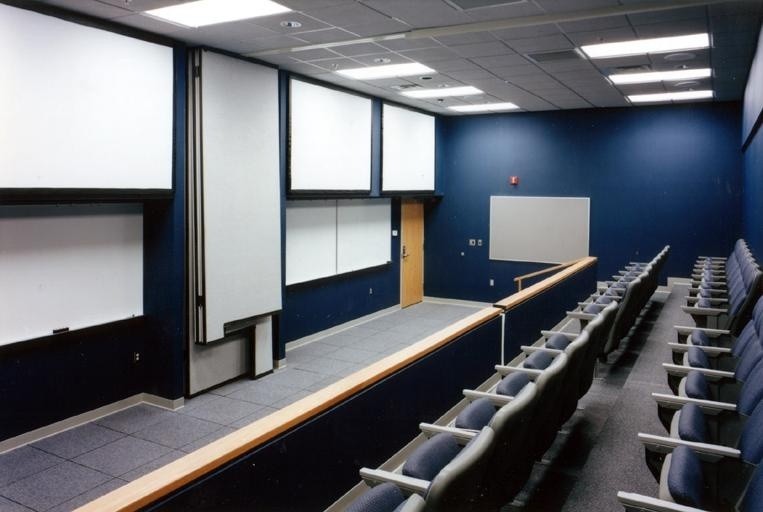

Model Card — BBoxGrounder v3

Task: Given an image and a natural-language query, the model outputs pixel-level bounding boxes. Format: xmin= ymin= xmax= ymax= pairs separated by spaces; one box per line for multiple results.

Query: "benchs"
xmin=565 ymin=245 xmax=675 ymax=352
xmin=680 ymin=238 xmax=763 ymax=327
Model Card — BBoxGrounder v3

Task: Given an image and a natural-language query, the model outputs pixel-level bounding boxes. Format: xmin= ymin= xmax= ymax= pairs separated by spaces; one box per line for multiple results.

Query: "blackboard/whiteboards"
xmin=0 ymin=201 xmax=145 ymax=348
xmin=285 ymin=197 xmax=392 ymax=288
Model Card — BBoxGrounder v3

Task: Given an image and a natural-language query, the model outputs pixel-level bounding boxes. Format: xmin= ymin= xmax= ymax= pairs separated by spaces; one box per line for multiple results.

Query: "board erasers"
xmin=52 ymin=326 xmax=70 ymax=334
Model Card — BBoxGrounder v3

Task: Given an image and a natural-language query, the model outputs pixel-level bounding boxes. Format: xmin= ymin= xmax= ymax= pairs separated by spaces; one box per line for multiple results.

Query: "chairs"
xmin=419 ymin=382 xmax=545 ymax=508
xmin=638 ymin=400 xmax=763 ymax=457
xmin=346 ymin=480 xmax=426 ymax=512
xmin=649 ymin=360 xmax=763 ymax=415
xmin=360 ymin=421 xmax=498 ymax=512
xmin=519 ymin=328 xmax=596 ymax=400
xmin=542 ymin=300 xmax=618 ymax=360
xmin=617 ymin=444 xmax=763 ymax=512
xmin=668 ymin=296 xmax=763 ymax=352
xmin=495 ymin=354 xmax=578 ymax=439
xmin=659 ymin=314 xmax=763 ymax=382
xmin=462 ymin=350 xmax=572 ymax=463
